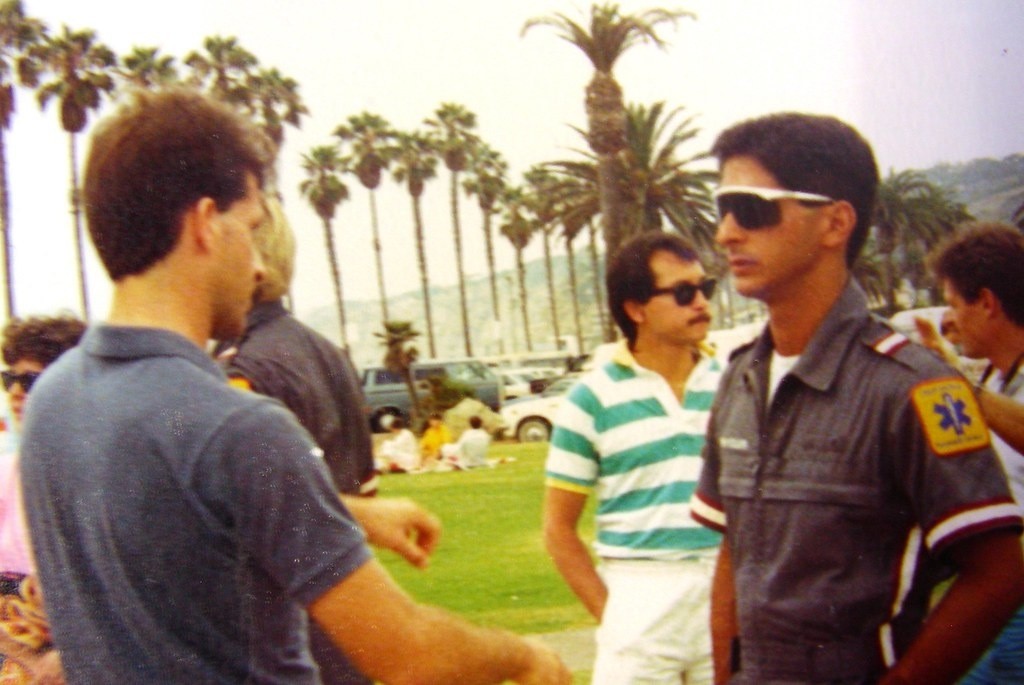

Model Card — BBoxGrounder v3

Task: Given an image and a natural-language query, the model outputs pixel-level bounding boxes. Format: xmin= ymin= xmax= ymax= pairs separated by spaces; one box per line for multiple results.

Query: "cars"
xmin=501 ymin=372 xmax=590 ymax=444
xmin=495 ymin=369 xmax=544 ymax=399
xmin=893 ymin=305 xmax=969 ymax=357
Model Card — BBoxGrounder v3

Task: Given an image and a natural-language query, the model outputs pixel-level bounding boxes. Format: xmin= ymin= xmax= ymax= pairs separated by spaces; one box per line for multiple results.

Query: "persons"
xmin=15 ymin=88 xmax=571 ymax=685
xmin=692 ymin=112 xmax=1024 ymax=685
xmin=0 ymin=319 xmax=85 ymax=595
xmin=217 ymin=190 xmax=377 ymax=685
xmin=543 ymin=232 xmax=724 ymax=685
xmin=380 ymin=414 xmax=489 ymax=466
xmin=916 ymin=220 xmax=1024 ymax=685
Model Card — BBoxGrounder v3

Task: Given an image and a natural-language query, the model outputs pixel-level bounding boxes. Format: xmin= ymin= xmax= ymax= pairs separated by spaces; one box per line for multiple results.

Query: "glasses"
xmin=713 ymin=185 xmax=837 ymax=231
xmin=1 ymin=371 xmax=38 ymax=394
xmin=641 ymin=279 xmax=717 ymax=307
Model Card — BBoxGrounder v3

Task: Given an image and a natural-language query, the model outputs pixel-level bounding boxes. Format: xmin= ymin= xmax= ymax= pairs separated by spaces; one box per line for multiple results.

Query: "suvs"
xmin=423 ymin=360 xmax=544 ymax=410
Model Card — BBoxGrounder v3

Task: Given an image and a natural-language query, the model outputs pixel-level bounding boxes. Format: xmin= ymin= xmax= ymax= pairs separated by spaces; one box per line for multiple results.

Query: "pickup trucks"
xmin=357 ymin=364 xmax=469 ymax=429
